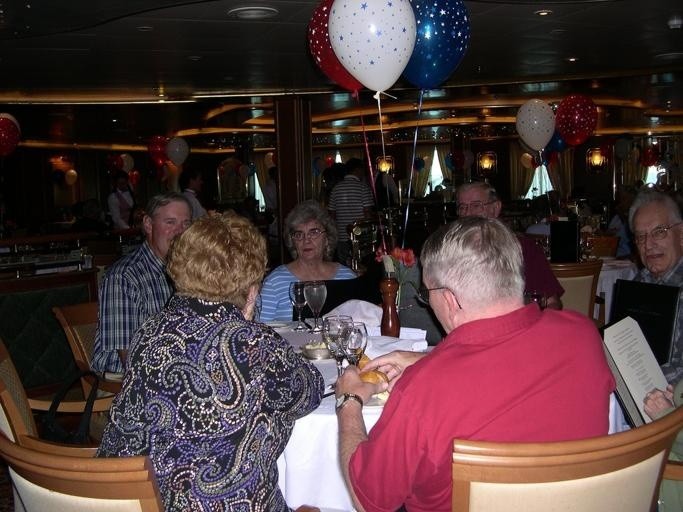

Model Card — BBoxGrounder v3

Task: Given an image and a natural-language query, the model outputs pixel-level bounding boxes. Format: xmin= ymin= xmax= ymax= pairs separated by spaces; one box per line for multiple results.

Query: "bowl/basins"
xmin=298 ymin=344 xmax=331 ymax=359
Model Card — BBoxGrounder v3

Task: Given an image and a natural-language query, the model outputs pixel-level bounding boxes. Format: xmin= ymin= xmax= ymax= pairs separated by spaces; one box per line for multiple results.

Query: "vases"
xmin=381 ymin=279 xmax=401 ymax=339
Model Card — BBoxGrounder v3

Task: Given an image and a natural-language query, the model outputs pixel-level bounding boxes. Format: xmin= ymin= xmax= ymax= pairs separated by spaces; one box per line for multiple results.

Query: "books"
xmin=596 ymin=316 xmax=670 ymax=428
xmin=608 ymin=279 xmax=680 ymax=368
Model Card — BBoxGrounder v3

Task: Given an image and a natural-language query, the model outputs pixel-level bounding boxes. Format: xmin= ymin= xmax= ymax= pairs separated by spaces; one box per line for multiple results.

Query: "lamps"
xmin=376 ymin=156 xmax=393 ymax=174
xmin=477 ymin=154 xmax=494 ymax=174
xmin=590 ymin=148 xmax=603 ymax=168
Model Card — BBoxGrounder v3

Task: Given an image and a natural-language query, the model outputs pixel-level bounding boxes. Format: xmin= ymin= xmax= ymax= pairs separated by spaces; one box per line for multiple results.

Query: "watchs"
xmin=335 ymin=392 xmax=364 ymax=413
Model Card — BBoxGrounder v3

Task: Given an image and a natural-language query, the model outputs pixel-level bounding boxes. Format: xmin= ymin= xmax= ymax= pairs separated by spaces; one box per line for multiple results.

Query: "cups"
xmin=323 ymin=315 xmax=354 ymax=377
xmin=289 ymin=280 xmax=307 ymax=331
xmin=303 ymin=281 xmax=327 ymax=334
xmin=338 ymin=322 xmax=369 ymax=367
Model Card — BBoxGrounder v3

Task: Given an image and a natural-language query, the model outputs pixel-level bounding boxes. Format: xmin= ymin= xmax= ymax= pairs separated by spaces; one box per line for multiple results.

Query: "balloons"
xmin=120 ymin=154 xmax=134 ymax=170
xmin=307 ymin=0 xmax=364 ymax=91
xmin=108 ymin=153 xmax=124 ymax=170
xmin=147 ymin=135 xmax=167 ymax=166
xmin=325 ymin=155 xmax=334 ymax=167
xmin=166 ymin=137 xmax=188 ymax=167
xmin=516 ymin=99 xmax=556 ymax=155
xmin=556 ymin=93 xmax=598 ymax=147
xmin=66 ymin=169 xmax=78 ymax=185
xmin=128 ymin=170 xmax=138 ymax=184
xmin=264 ymin=152 xmax=275 ymax=167
xmin=403 ymin=0 xmax=471 ymax=88
xmin=0 ymin=112 xmax=20 ymax=156
xmin=328 ymin=0 xmax=417 ymax=94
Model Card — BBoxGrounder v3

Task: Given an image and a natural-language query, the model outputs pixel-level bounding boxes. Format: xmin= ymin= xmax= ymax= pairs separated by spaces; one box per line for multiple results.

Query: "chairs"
xmin=451 ymin=406 xmax=683 ymax=512
xmin=0 ymin=431 xmax=164 ymax=512
xmin=547 ymin=261 xmax=606 ymax=332
xmin=0 ymin=341 xmax=117 ymax=458
xmin=662 ymin=460 xmax=683 ymax=512
xmin=53 ymin=303 xmax=124 ymax=424
xmin=581 ymin=234 xmax=619 ymax=257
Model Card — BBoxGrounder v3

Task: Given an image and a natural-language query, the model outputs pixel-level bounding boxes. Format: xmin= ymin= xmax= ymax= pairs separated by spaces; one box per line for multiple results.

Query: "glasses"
xmin=458 ymin=198 xmax=497 ymax=217
xmin=290 ymin=228 xmax=326 ymax=241
xmin=416 ymin=287 xmax=462 ymax=310
xmin=632 ymin=221 xmax=680 ymax=245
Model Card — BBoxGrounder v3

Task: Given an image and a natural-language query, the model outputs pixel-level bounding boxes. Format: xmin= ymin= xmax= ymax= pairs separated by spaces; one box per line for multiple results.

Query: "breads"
xmin=358 ymin=368 xmax=388 ymax=384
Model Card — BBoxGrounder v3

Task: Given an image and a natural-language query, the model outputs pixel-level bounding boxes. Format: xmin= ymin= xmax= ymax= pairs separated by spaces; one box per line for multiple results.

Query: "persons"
xmin=457 ymin=182 xmax=565 ymax=310
xmin=372 ymin=165 xmax=398 ymax=205
xmin=94 ymin=211 xmax=325 ymax=512
xmin=108 ymin=172 xmax=134 ymax=230
xmin=642 ymin=379 xmax=683 ymax=511
xmin=91 ymin=191 xmax=193 ymax=375
xmin=260 ymin=203 xmax=358 ymax=323
xmin=328 ymin=158 xmax=374 ymax=255
xmin=177 ymin=168 xmax=206 ymax=219
xmin=334 ymin=215 xmax=617 ymax=512
xmin=627 ymin=191 xmax=683 ymax=381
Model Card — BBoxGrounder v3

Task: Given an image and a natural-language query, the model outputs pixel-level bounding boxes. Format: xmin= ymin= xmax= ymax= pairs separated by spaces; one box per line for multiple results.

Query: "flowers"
xmin=376 ymin=247 xmax=414 ymax=313
